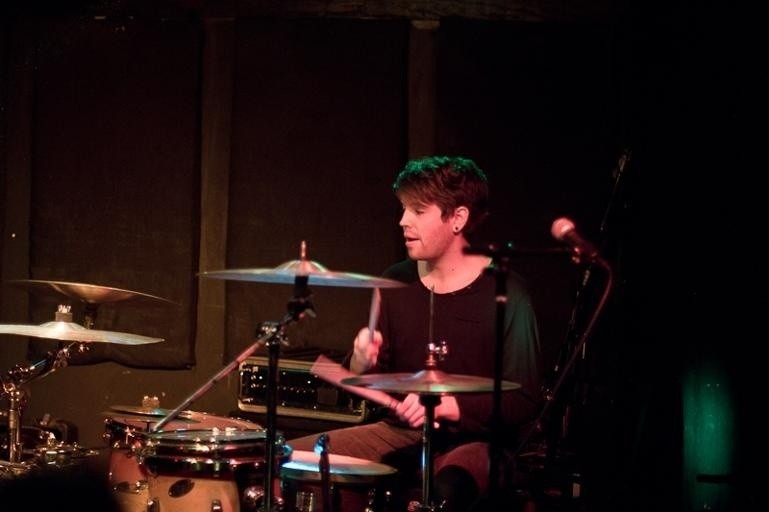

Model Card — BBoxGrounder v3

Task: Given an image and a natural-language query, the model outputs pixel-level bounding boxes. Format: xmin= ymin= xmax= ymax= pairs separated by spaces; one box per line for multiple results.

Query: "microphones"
xmin=551 ymin=217 xmax=613 ymax=272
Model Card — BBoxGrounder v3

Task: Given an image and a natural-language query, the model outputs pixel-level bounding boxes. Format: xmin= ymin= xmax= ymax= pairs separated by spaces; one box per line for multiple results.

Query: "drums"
xmin=140 ymin=428 xmax=287 ymax=510
xmin=100 ymin=406 xmax=262 ymax=511
xmin=282 ymin=472 xmax=394 ymax=512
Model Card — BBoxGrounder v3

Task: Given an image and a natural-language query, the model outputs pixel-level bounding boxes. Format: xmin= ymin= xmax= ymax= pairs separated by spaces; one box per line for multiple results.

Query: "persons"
xmin=281 ymin=156 xmax=543 ymax=512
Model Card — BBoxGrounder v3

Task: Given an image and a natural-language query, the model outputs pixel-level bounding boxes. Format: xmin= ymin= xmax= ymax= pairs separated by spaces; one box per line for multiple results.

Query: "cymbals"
xmin=195 ymin=258 xmax=410 ymax=289
xmin=0 ymin=305 xmax=165 ymax=348
xmin=338 ymin=367 xmax=522 ymax=396
xmin=279 ymin=448 xmax=400 ymax=477
xmin=106 ymin=403 xmax=193 ymax=419
xmin=4 ymin=277 xmax=185 ymax=308
